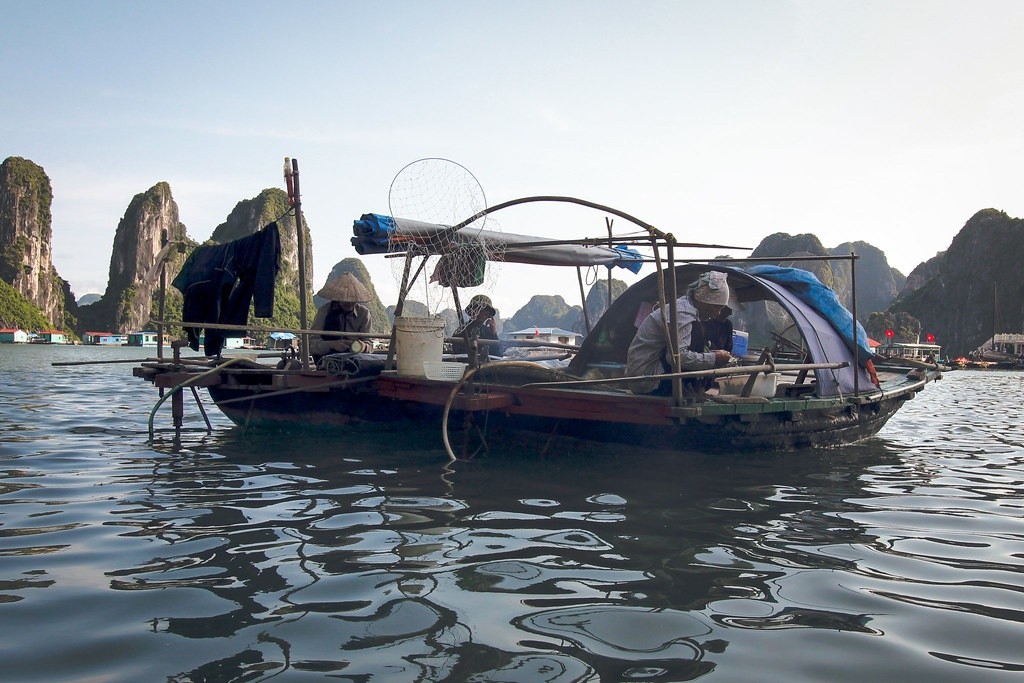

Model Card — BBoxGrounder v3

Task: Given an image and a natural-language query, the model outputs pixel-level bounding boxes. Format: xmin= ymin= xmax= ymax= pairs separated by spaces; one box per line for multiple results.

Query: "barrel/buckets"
xmin=742 ymin=355 xmax=765 ymax=374
xmin=394 ymin=316 xmax=446 ymax=378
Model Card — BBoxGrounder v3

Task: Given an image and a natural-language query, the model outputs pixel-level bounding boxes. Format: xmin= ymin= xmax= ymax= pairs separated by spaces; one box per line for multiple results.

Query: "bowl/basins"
xmin=714 ymin=372 xmax=781 ymax=398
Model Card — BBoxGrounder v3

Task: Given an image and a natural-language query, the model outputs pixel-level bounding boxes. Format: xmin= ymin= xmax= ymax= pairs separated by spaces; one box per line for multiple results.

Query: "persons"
xmin=451 ymin=295 xmax=503 ymax=363
xmin=310 ymin=273 xmax=374 ymax=371
xmin=625 ymin=270 xmax=746 ymax=404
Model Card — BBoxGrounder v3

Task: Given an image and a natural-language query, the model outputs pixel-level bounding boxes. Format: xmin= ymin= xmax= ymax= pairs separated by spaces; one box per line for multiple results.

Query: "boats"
xmin=52 ymin=159 xmax=952 ymax=457
xmin=874 ymin=321 xmax=952 ymax=372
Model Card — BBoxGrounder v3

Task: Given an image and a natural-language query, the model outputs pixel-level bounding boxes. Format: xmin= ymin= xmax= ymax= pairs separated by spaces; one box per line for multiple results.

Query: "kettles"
xmin=300 ymin=356 xmax=317 ymax=371
xmin=277 ymin=344 xmax=302 ymax=370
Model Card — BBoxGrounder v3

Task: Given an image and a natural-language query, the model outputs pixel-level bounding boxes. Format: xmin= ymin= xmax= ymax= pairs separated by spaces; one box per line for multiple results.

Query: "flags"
xmin=927 ymin=333 xmax=935 ymax=342
xmin=534 ymin=330 xmax=539 ymax=336
xmin=885 ymin=330 xmax=894 ymax=338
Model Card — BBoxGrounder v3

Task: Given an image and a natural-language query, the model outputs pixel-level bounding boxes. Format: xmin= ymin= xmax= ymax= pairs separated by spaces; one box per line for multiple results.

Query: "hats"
xmin=465 ymin=295 xmax=495 ymax=317
xmin=317 ymin=271 xmax=374 ymax=303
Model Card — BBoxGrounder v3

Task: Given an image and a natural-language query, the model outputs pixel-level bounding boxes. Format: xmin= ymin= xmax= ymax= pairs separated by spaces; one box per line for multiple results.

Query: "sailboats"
xmin=968 ymin=281 xmax=1024 ymax=368
